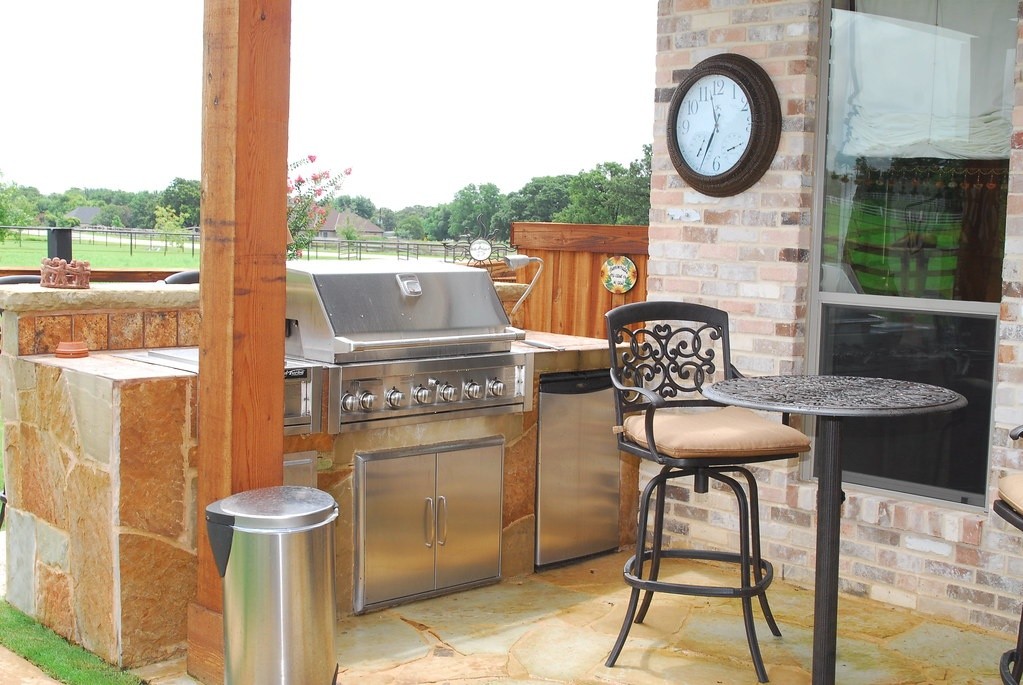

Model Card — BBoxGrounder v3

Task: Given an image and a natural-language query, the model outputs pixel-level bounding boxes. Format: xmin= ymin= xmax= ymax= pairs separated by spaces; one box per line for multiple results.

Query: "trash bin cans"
xmin=204 ymin=485 xmax=340 ymax=685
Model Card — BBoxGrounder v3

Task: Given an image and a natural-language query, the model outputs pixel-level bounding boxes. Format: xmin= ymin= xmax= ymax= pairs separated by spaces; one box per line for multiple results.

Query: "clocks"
xmin=663 ymin=49 xmax=787 ymax=198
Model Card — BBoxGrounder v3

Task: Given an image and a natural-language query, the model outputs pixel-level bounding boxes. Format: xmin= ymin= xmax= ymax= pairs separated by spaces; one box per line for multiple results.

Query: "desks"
xmin=701 ymin=373 xmax=973 ymax=685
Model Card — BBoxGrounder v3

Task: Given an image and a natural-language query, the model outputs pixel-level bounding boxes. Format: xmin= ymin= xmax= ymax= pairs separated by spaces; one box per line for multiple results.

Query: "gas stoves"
xmin=286 ymin=256 xmax=538 ymax=433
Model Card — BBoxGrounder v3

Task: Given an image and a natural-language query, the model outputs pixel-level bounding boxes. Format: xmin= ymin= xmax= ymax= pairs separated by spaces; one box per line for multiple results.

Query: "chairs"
xmin=601 ymin=300 xmax=788 ymax=685
xmin=991 ymin=427 xmax=1023 ymax=685
